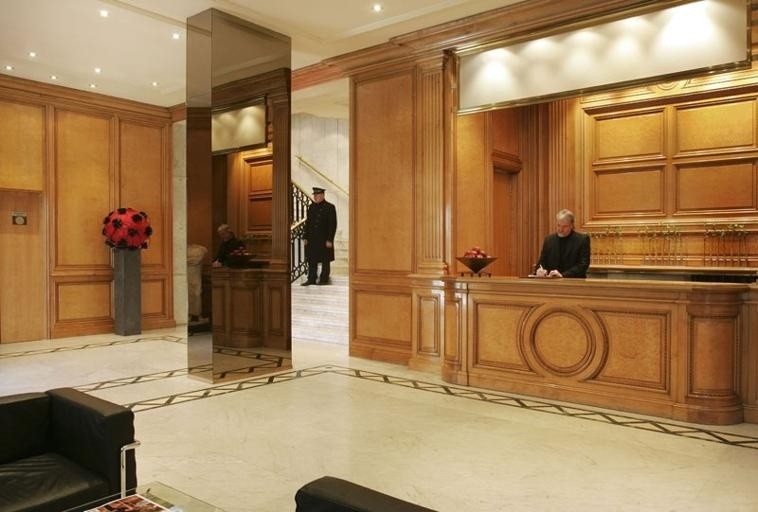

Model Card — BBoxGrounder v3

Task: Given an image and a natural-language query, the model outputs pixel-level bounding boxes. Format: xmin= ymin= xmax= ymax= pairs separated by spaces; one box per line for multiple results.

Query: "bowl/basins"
xmin=455 ymin=257 xmax=500 ymax=274
xmin=224 ymin=255 xmax=256 ymax=267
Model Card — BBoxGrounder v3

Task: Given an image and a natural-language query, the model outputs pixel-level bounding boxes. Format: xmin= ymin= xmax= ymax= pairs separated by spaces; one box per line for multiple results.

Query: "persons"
xmin=299 ymin=186 xmax=337 ymax=287
xmin=211 ymin=224 xmax=245 ymax=267
xmin=532 ymin=207 xmax=591 ymax=280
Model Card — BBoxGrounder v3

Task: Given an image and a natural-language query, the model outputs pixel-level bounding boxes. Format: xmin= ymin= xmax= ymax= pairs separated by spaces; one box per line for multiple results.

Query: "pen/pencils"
xmin=539 ymin=264 xmax=546 ymax=277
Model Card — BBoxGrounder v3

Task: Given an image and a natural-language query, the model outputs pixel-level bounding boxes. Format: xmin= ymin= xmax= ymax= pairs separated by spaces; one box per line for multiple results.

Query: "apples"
xmin=229 ymin=246 xmax=249 ymax=256
xmin=464 ymin=247 xmax=491 ymax=259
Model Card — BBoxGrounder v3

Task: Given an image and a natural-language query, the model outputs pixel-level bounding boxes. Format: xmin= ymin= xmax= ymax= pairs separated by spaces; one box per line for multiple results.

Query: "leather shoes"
xmin=300 ymin=280 xmax=329 ymax=286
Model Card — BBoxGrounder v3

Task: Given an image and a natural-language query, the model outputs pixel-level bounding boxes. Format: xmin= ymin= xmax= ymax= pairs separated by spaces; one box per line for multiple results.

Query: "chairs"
xmin=295 ymin=476 xmax=437 ymax=512
xmin=0 ymin=388 xmax=136 ymax=512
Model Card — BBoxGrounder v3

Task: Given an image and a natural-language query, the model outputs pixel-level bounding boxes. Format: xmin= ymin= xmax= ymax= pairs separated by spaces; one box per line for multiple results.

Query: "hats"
xmin=311 ymin=187 xmax=326 ymax=195
